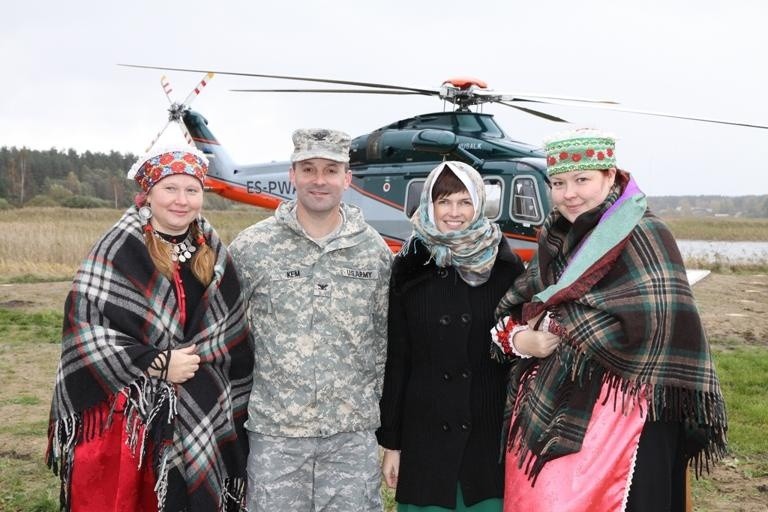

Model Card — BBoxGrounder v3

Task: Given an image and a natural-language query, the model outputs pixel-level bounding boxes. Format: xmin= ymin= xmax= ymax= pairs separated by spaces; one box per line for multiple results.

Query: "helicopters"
xmin=119 ymin=64 xmax=768 ymax=269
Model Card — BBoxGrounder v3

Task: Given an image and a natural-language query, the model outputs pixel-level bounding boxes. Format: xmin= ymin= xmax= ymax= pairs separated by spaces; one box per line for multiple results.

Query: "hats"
xmin=544 ymin=136 xmax=616 ymax=177
xmin=126 ymin=144 xmax=209 ymax=196
xmin=290 ymin=129 xmax=351 ymax=163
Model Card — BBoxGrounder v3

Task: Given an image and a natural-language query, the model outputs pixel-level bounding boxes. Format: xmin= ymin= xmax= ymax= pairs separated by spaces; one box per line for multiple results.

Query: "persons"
xmin=375 ymin=160 xmax=527 ymax=512
xmin=487 ymin=136 xmax=728 ymax=512
xmin=229 ymin=128 xmax=395 ymax=512
xmin=44 ymin=146 xmax=256 ymax=512
